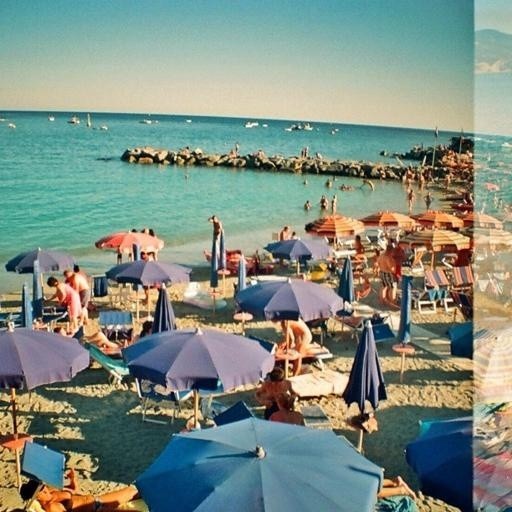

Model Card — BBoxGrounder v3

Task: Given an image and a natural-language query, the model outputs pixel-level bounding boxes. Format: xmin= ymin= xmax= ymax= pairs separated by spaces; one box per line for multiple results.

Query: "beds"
xmin=274 ymin=343 xmax=334 ymax=372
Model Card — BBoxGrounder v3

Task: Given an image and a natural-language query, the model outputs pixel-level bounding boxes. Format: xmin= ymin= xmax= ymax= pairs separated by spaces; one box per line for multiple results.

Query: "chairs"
xmin=82 ymin=342 xmax=129 ymax=387
xmin=329 ymin=310 xmax=392 ymax=344
xmin=424 ymin=268 xmax=452 ymax=291
xmin=449 ymin=290 xmax=512 ymax=330
xmin=486 ymin=272 xmax=511 ymax=300
xmin=191 ymin=332 xmax=277 ymax=414
xmin=450 ymin=266 xmax=477 ymax=300
xmin=411 ymin=289 xmax=443 ymax=314
xmin=440 ymin=290 xmax=460 ymax=314
xmin=135 ymin=377 xmax=194 ymax=427
xmin=89 ymin=275 xmax=114 ymax=310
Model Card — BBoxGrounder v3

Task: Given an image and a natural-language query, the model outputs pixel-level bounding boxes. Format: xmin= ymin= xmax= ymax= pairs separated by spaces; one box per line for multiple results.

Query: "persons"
xmin=408 ymin=190 xmax=415 ymax=207
xmin=445 ymin=173 xmax=449 ymax=189
xmin=132 ymin=227 xmax=155 ymax=236
xmin=331 ymin=196 xmax=337 ymax=210
xmin=377 ymin=474 xmax=415 ymax=502
xmin=424 ymin=192 xmax=433 ymax=209
xmin=348 ymin=233 xmax=368 ymax=272
xmin=20 ymin=479 xmax=139 ymax=511
xmin=269 ymin=393 xmax=304 ymax=426
xmin=340 ymin=183 xmax=352 ymax=189
xmin=255 ymin=368 xmax=295 ymax=420
xmin=274 ymin=314 xmax=329 ymax=377
xmin=90 ymin=320 xmax=153 ymax=349
xmin=326 ymin=177 xmax=331 ymax=186
xmin=257 ymin=149 xmax=264 ymax=157
xmin=292 ymin=232 xmax=298 ymax=240
xmin=302 ymin=146 xmax=308 ymax=157
xmin=63 ymin=271 xmax=91 ymax=311
xmin=229 ymin=141 xmax=242 ymax=159
xmin=43 ymin=276 xmax=81 ymax=332
xmin=378 ymin=244 xmax=398 ymax=306
xmin=182 ymin=145 xmax=190 ymax=156
xmin=320 ymin=195 xmax=325 ymax=206
xmin=402 ymin=167 xmax=426 ymax=192
xmin=141 ymin=250 xmax=151 ymax=306
xmin=207 ymin=215 xmax=222 ymax=240
xmin=392 ymin=240 xmax=405 ymax=274
xmin=279 ymin=225 xmax=290 ymax=268
xmin=376 ymin=168 xmax=386 ymax=178
xmin=304 ymin=178 xmax=308 ymax=184
xmin=315 ymin=151 xmax=321 ymax=162
xmin=304 ymin=200 xmax=312 ymax=208
xmin=71 ymin=263 xmax=84 ymax=275
xmin=333 ymin=175 xmax=337 ymax=181
xmin=363 ymin=179 xmax=373 ymax=187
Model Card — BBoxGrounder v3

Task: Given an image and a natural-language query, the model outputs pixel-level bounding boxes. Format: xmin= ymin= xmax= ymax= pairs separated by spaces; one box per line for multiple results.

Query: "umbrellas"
xmin=105 ymin=260 xmax=191 ymax=318
xmin=128 ymin=415 xmax=384 ymax=510
xmin=402 ymin=229 xmax=471 ymax=269
xmin=1 ymin=322 xmax=91 ymax=440
xmin=407 ymin=414 xmax=473 ymax=507
xmin=475 ymin=231 xmax=512 ymax=253
xmin=237 ymin=258 xmax=245 ymax=312
xmin=360 ymin=211 xmax=420 ymax=229
xmin=305 ymin=215 xmax=364 ymax=237
xmin=474 ymin=324 xmax=511 ymax=399
xmin=4 ymin=247 xmax=77 ymax=320
xmin=448 ymin=318 xmax=473 ymax=362
xmin=120 ymin=327 xmax=275 ymax=426
xmin=131 ymin=242 xmax=142 ymax=290
xmin=210 ymin=237 xmax=218 ymax=324
xmin=233 ymin=277 xmax=344 ymax=379
xmin=401 ymin=277 xmax=411 ymax=345
xmin=342 ymin=324 xmax=388 ymax=450
xmin=410 ymin=210 xmax=464 ymax=230
xmin=473 ymin=211 xmax=502 ymax=228
xmin=150 ymin=289 xmax=174 ymax=334
xmin=264 ymin=237 xmax=329 ymax=273
xmin=220 ymin=233 xmax=226 ymax=271
xmin=337 ymin=259 xmax=355 ymax=316
xmin=95 ymin=234 xmax=165 ymax=253
xmin=21 ymin=282 xmax=35 ymax=328
xmin=32 ymin=267 xmax=42 ymax=316
xmin=477 ymin=406 xmax=512 ymax=511
